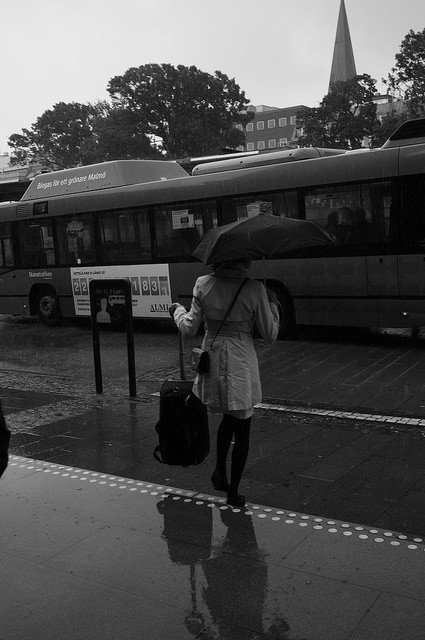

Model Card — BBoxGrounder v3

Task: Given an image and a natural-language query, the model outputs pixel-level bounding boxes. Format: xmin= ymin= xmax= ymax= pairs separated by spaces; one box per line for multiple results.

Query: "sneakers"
xmin=212 ymin=468 xmax=228 ymax=491
xmin=226 ymin=493 xmax=245 ymax=505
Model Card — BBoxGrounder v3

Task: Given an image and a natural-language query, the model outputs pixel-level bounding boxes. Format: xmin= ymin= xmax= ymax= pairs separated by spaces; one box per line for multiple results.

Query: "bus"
xmin=0 ymin=119 xmax=425 ymax=337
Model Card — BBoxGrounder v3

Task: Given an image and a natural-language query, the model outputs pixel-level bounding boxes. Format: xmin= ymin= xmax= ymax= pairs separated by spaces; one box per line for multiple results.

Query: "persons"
xmin=170 ymin=262 xmax=279 ymax=505
xmin=183 ymin=505 xmax=288 ymax=639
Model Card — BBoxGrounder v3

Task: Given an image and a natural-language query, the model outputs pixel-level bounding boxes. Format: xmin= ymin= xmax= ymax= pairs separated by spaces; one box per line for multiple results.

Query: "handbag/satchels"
xmin=189 ymin=348 xmax=210 ymax=373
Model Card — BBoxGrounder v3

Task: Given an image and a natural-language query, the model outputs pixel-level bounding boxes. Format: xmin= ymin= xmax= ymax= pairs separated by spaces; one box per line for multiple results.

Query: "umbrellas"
xmin=196 ymin=209 xmax=338 ymax=291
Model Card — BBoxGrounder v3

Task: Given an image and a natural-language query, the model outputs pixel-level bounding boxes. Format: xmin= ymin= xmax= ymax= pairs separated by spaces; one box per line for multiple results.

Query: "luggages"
xmin=154 ymin=306 xmax=210 ymax=466
